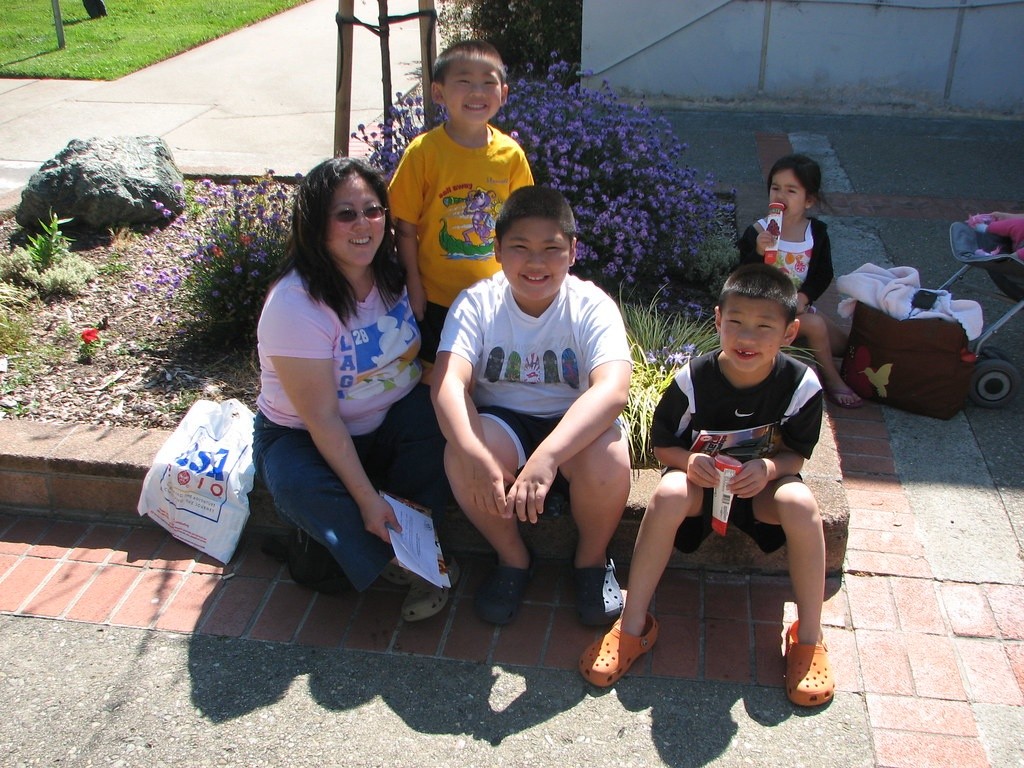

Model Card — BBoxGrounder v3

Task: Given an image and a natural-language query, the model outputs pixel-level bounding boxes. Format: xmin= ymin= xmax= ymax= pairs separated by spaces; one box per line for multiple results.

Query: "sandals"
xmin=572 ymin=558 xmax=623 ymax=627
xmin=785 ymin=621 xmax=833 ymax=707
xmin=579 ymin=611 xmax=660 ymax=687
xmin=400 ymin=555 xmax=460 ymax=622
xmin=473 ymin=564 xmax=537 ymax=627
xmin=377 ymin=562 xmax=411 ymax=585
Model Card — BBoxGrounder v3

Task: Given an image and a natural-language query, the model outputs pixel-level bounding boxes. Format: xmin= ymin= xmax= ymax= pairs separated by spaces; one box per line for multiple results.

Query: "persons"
xmin=429 ymin=186 xmax=633 ymax=627
xmin=967 ymin=213 xmax=1024 ymax=261
xmin=738 ymin=153 xmax=869 ymax=409
xmin=579 ymin=261 xmax=837 ymax=708
xmin=384 ymin=39 xmax=538 ymax=365
xmin=252 ymin=157 xmax=453 ymax=623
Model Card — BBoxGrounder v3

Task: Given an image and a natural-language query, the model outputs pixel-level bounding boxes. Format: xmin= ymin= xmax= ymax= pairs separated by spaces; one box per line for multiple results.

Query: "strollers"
xmin=935 ymin=210 xmax=1024 ymax=407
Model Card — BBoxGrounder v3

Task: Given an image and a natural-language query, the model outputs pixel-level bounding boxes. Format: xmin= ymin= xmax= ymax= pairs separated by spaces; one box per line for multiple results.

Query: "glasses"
xmin=325 ymin=207 xmax=389 ymax=222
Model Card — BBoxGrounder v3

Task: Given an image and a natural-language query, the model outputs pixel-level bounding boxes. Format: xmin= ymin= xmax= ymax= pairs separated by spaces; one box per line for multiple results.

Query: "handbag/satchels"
xmin=136 ymin=395 xmax=257 ymax=565
xmin=841 ymin=294 xmax=974 ymax=420
xmin=261 ymin=528 xmax=357 ymax=592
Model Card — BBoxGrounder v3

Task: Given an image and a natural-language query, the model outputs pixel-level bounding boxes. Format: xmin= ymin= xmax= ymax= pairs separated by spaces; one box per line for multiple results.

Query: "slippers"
xmin=825 ymin=384 xmax=865 ymax=408
